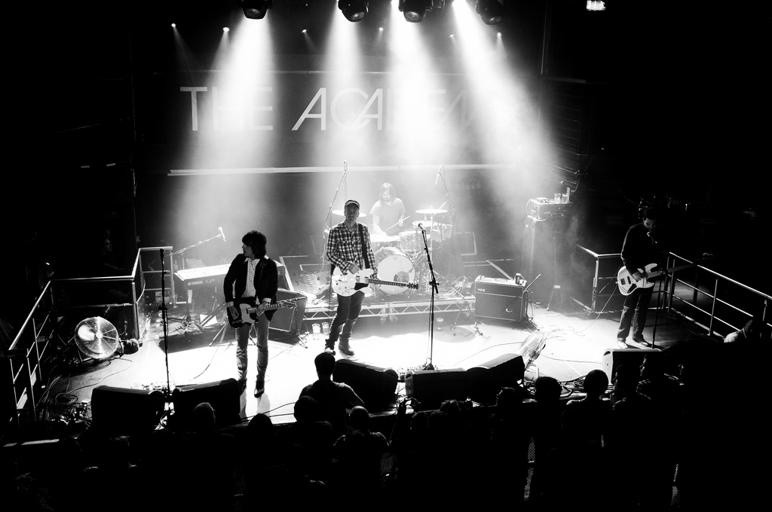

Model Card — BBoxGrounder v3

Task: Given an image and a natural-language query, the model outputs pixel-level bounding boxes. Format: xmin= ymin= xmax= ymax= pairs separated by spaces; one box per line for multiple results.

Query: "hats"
xmin=345 ymin=200 xmax=360 ymax=209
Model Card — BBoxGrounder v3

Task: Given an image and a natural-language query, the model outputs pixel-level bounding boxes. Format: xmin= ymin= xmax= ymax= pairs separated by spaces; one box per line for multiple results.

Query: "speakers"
xmin=475 ymin=278 xmax=527 ymax=322
xmin=269 ymin=289 xmax=306 ymax=339
xmin=91 ymin=384 xmax=166 ymax=432
xmin=466 ymin=352 xmax=525 ymax=402
xmin=333 ymin=359 xmax=399 ymax=411
xmin=601 ymin=348 xmax=665 ymax=384
xmin=523 ymin=198 xmax=576 ymax=302
xmin=172 ymin=376 xmax=246 ymax=425
xmin=411 ymin=367 xmax=466 ymax=413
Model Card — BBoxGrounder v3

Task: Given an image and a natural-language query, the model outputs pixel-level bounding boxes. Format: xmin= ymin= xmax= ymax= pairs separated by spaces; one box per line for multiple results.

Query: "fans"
xmin=71 ymin=314 xmax=120 ymax=362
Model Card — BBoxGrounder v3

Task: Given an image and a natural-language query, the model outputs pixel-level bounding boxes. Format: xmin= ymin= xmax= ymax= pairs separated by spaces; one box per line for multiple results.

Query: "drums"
xmin=372 ymin=233 xmax=400 ymax=251
xmin=371 ymin=247 xmax=415 ymax=295
xmin=413 ymin=220 xmax=442 ymax=239
xmin=400 ymin=229 xmax=427 ymax=252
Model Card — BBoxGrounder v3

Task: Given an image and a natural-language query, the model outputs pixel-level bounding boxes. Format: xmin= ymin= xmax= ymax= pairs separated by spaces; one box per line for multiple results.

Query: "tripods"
xmin=209 ymin=317 xmax=256 ymax=347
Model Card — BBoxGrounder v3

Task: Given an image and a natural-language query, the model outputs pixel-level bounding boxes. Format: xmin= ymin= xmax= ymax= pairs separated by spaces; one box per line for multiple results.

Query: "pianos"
xmin=172 ymin=258 xmax=287 ymax=290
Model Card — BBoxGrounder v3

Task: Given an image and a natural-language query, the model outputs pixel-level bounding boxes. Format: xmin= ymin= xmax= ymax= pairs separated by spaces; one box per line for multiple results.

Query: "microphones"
xmin=434 ymin=168 xmax=442 ymax=186
xmin=218 ymin=225 xmax=225 ymax=240
xmin=344 ymin=161 xmax=348 ymax=172
xmin=647 ymin=230 xmax=659 ymax=246
xmin=159 ymin=248 xmax=164 ymax=267
xmin=418 ymin=221 xmax=427 ymax=234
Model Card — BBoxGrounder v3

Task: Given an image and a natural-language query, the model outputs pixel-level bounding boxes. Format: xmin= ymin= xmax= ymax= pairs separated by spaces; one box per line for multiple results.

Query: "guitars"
xmin=226 ymin=298 xmax=299 ymax=327
xmin=332 ymin=262 xmax=419 ymax=297
xmin=617 ymin=252 xmax=715 ymax=297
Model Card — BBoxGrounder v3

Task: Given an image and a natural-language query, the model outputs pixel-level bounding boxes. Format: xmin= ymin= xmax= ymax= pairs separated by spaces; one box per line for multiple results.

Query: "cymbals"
xmin=416 ymin=208 xmax=448 ymax=216
xmin=332 ymin=210 xmax=368 ymax=218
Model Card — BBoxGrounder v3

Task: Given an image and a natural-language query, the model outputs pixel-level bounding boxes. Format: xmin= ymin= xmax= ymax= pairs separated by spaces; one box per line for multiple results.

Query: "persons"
xmin=367 ymin=180 xmax=408 ymax=238
xmin=51 ymin=316 xmax=771 ymax=511
xmin=614 ymin=211 xmax=663 ymax=348
xmin=321 ymin=198 xmax=379 ymax=357
xmin=222 ymin=230 xmax=280 ymax=398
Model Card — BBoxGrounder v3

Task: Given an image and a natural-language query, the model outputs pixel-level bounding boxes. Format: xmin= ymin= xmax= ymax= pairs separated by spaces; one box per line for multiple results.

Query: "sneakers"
xmin=341 ymin=350 xmax=353 ymax=355
xmin=254 ymin=388 xmax=264 ymax=397
xmin=325 ymin=348 xmax=336 ymax=356
